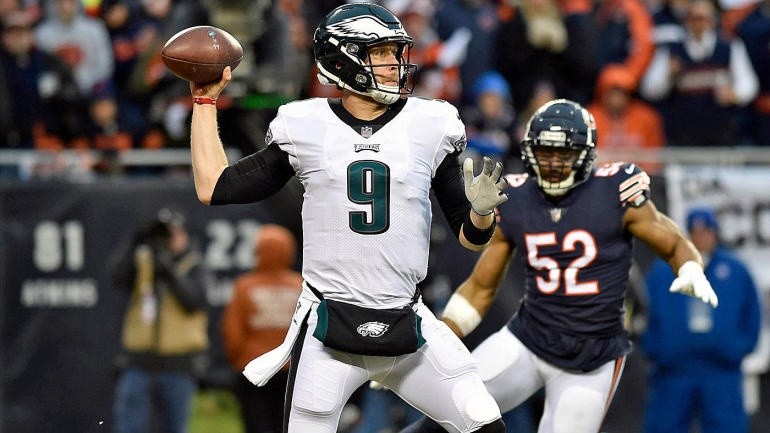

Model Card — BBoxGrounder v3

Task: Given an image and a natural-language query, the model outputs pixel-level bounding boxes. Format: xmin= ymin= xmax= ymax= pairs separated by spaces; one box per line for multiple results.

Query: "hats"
xmin=687 ymin=209 xmax=719 ymax=231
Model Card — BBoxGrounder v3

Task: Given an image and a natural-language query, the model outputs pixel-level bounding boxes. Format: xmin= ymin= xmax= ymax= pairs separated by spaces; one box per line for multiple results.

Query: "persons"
xmin=103 ymin=214 xmax=212 ymax=433
xmin=220 ymin=223 xmax=305 ymax=432
xmin=633 ymin=208 xmax=760 ymax=432
xmin=190 ymin=4 xmax=511 ymax=433
xmin=0 ymin=0 xmax=770 ymax=176
xmin=440 ymin=100 xmax=717 ymax=433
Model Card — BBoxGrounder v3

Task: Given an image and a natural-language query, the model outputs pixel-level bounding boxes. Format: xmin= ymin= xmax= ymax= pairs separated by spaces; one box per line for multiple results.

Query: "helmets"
xmin=519 ymin=99 xmax=597 ymax=197
xmin=313 ymin=3 xmax=418 ymax=105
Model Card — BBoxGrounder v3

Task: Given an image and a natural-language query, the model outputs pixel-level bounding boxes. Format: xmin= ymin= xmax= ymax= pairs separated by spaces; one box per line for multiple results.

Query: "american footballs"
xmin=162 ymin=26 xmax=245 ymax=82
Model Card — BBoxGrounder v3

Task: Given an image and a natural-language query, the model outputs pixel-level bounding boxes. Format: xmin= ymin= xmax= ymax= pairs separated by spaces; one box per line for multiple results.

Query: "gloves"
xmin=669 ymin=261 xmax=718 ymax=308
xmin=463 ymin=156 xmax=509 ymax=216
